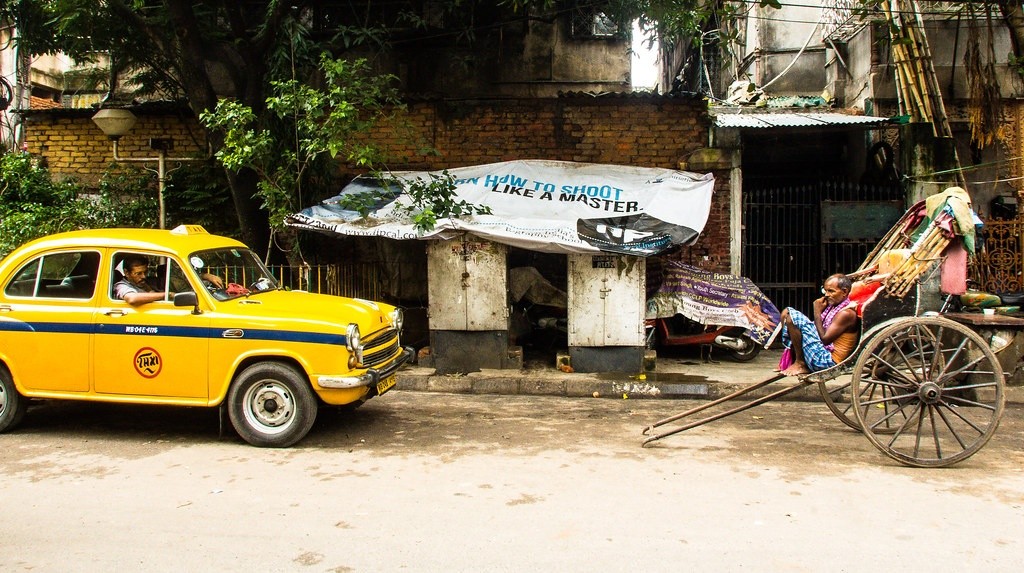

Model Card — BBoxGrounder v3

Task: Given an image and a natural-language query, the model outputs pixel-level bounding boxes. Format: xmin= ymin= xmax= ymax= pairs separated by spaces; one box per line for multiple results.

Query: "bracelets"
xmin=169 ymin=292 xmax=172 ymax=298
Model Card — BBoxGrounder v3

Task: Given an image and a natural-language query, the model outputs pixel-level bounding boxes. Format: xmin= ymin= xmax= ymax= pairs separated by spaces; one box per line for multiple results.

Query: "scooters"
xmin=638 ymin=257 xmax=765 ymax=364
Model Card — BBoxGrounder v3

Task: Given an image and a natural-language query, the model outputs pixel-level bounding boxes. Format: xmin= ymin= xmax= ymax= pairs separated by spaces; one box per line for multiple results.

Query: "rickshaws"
xmin=641 ymin=181 xmax=1013 ymax=469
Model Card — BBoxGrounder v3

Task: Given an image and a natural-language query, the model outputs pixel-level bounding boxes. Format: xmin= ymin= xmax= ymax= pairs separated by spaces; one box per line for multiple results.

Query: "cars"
xmin=1 ymin=223 xmax=415 ymax=448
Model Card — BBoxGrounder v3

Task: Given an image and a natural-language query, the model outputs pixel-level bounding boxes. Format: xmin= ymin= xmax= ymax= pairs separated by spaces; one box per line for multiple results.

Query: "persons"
xmin=113 ymin=254 xmax=176 ymax=306
xmin=170 ymin=257 xmax=223 ymax=294
xmin=773 ymin=273 xmax=859 ymax=377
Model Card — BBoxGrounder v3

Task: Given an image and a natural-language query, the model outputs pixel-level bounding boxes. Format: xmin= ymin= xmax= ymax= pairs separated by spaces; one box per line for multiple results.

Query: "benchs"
xmin=843 ymin=249 xmax=912 ymax=318
xmin=9 ymin=275 xmax=94 ymax=298
